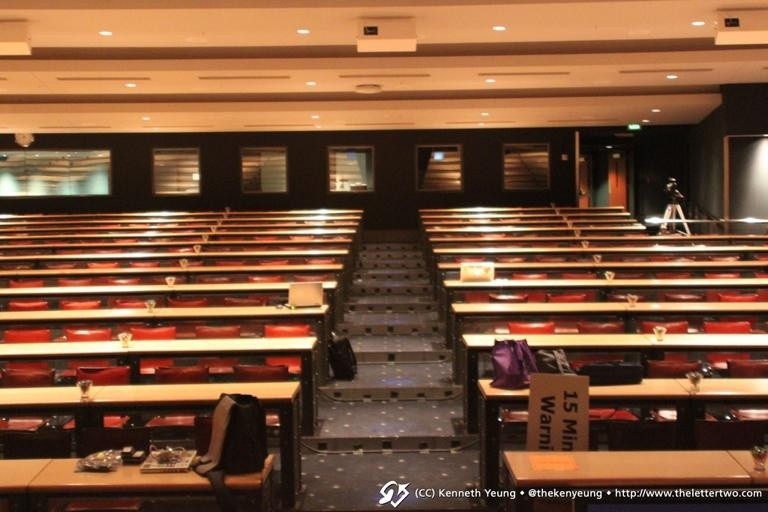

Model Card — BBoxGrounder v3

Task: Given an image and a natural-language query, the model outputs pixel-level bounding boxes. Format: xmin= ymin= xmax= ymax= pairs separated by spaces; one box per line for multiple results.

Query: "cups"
xmin=580 ymin=241 xmax=590 ymax=250
xmin=551 ymin=204 xmax=581 ymax=237
xmin=626 ymin=294 xmax=639 ymax=307
xmin=118 ymin=332 xmax=133 ymax=348
xmin=76 ymin=380 xmax=92 ymax=398
xmin=604 ymin=271 xmax=615 ymax=284
xmin=145 ymin=300 xmax=156 ymax=313
xmin=653 ymin=327 xmax=668 ymax=341
xmin=685 ymin=372 xmax=703 ymax=392
xmin=202 ymin=206 xmax=231 ymax=242
xmin=178 ymin=258 xmax=188 ymax=269
xmin=750 ymin=445 xmax=768 ymax=471
xmin=593 ymin=254 xmax=602 ymax=264
xmin=193 ymin=245 xmax=202 ymax=254
xmin=165 ymin=276 xmax=177 ymax=288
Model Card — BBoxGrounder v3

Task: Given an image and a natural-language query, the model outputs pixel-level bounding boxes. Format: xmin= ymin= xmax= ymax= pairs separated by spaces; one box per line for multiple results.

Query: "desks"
xmin=417 ymin=206 xmax=768 ymax=512
xmin=0 ymin=209 xmax=364 ymax=512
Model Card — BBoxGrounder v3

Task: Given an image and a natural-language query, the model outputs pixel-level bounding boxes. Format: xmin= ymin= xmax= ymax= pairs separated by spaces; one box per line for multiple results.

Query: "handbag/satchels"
xmin=490 ymin=339 xmax=644 ymax=389
xmin=191 ymin=393 xmax=267 ymax=511
xmin=328 ymin=332 xmax=357 ymax=381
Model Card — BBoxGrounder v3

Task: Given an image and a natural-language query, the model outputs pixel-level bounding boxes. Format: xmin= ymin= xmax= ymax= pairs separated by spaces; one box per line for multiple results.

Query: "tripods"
xmin=658 ymin=197 xmax=691 ymax=237
